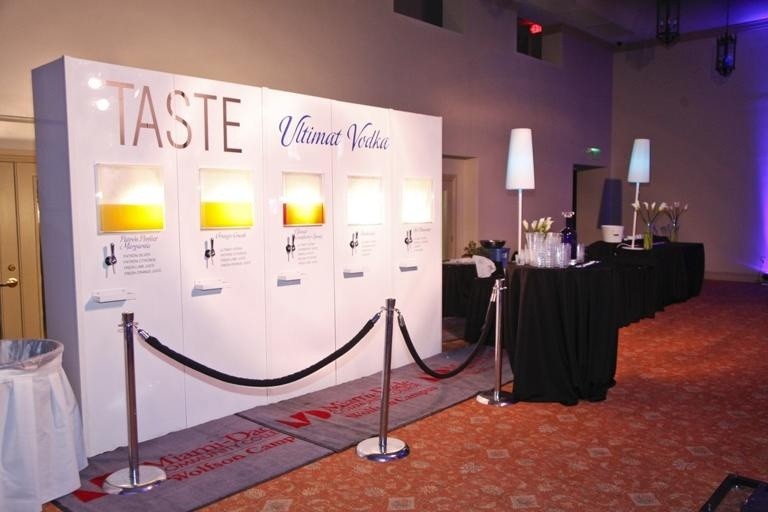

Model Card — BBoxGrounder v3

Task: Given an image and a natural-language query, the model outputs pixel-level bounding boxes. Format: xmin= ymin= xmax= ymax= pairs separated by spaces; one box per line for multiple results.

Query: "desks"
xmin=591 ymin=240 xmax=705 ymax=332
xmin=507 ymin=260 xmax=621 ymax=404
xmin=442 ymin=257 xmax=518 ymax=320
xmin=466 ymin=277 xmax=509 ymax=348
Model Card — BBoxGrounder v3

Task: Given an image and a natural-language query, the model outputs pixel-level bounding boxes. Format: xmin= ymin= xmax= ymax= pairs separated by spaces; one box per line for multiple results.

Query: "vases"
xmin=672 ymin=225 xmax=679 ymax=242
xmin=644 ymin=230 xmax=653 ymax=250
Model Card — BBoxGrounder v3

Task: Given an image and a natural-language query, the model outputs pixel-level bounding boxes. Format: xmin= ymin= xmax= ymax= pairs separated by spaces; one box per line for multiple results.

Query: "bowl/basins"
xmin=0 ymin=339 xmax=63 ymax=372
xmin=480 ymin=240 xmax=505 ymax=248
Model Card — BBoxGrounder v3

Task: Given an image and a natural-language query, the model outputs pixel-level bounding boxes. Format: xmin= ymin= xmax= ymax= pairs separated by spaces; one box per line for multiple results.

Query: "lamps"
xmin=622 ymin=138 xmax=652 ymax=251
xmin=656 ymin=1 xmax=681 ymax=46
xmin=506 ymin=128 xmax=536 ymax=263
xmin=716 ymin=0 xmax=738 ymax=77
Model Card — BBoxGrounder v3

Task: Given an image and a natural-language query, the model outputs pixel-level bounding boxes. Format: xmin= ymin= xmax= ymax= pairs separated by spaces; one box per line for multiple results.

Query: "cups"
xmin=525 ymin=231 xmax=571 ymax=268
xmin=576 ymin=239 xmax=585 ymax=262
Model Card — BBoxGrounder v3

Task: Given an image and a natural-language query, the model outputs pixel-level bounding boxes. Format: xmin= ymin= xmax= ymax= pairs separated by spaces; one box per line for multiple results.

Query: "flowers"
xmin=523 ymin=216 xmax=552 ymax=235
xmin=632 ymin=200 xmax=668 ymax=226
xmin=664 ymin=201 xmax=689 ymax=225
